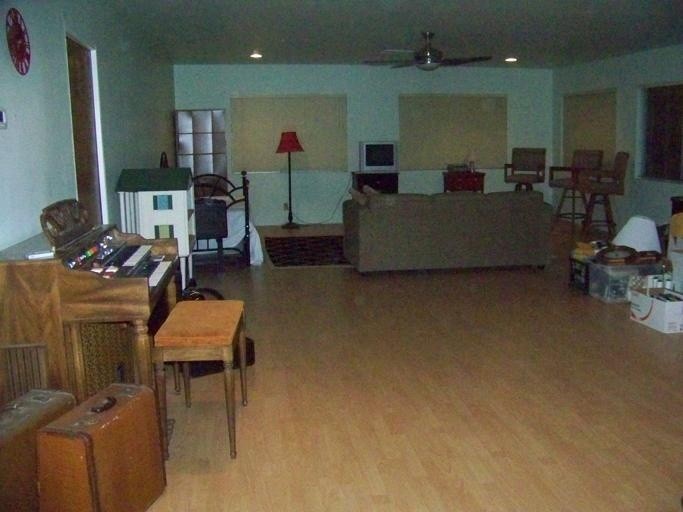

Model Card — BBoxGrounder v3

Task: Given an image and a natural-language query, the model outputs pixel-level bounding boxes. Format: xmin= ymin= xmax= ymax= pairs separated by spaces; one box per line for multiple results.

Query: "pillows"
xmin=346 ymin=186 xmax=368 ymax=206
xmin=360 ymin=183 xmax=379 ymax=196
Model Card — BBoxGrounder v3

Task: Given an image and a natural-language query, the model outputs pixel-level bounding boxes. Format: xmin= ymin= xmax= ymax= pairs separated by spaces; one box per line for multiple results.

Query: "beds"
xmin=154 ymin=148 xmax=265 ymax=272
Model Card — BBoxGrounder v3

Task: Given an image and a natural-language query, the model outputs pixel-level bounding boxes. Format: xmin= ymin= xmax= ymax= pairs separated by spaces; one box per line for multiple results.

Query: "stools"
xmin=153 ymin=299 xmax=250 ymax=458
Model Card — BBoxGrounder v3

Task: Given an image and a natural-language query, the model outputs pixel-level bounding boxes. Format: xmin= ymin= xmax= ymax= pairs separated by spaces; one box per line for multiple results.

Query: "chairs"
xmin=577 ymin=148 xmax=632 ymax=242
xmin=545 ymin=145 xmax=606 ymax=232
xmin=499 ymin=141 xmax=550 ymax=195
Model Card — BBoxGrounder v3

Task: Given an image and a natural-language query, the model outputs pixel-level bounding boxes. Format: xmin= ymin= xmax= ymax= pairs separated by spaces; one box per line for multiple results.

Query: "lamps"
xmin=609 ymin=213 xmax=662 ymax=254
xmin=272 ymin=130 xmax=307 ymax=231
xmin=415 ymin=61 xmax=441 ymax=71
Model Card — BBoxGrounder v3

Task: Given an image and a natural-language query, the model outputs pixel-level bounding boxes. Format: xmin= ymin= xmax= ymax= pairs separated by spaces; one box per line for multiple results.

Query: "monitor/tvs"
xmin=359 ymin=142 xmax=399 ymax=172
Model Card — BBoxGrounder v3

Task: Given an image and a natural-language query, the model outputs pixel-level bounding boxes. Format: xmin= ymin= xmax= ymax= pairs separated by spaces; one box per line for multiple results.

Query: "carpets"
xmin=261 ymin=233 xmax=352 ymax=271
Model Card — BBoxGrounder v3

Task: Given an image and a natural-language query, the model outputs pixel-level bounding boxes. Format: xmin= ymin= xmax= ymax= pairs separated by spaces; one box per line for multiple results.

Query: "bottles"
xmin=158 ymin=151 xmax=168 ymax=170
xmin=469 ymin=161 xmax=474 ymax=172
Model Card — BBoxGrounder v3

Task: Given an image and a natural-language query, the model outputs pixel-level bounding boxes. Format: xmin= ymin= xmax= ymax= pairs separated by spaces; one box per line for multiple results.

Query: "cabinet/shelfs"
xmin=439 ymin=168 xmax=488 ymax=196
xmin=347 ymin=169 xmax=402 ymax=197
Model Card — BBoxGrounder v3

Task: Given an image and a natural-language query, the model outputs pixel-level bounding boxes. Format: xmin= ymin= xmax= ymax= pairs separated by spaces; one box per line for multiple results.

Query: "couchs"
xmin=340 ymin=190 xmax=553 ymax=277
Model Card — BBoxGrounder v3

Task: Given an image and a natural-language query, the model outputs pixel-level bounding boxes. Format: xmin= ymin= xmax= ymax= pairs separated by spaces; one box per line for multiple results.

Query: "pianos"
xmin=0 ymin=223 xmax=180 ymax=406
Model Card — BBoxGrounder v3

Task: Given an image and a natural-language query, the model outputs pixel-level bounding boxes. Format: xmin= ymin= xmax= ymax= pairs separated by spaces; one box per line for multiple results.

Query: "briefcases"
xmin=36 ymin=382 xmax=167 ymax=512
xmin=0 ymin=382 xmax=79 ymax=512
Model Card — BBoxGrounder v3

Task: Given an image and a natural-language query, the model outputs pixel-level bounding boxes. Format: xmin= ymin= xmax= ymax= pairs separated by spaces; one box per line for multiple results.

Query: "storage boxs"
xmin=628 ymin=286 xmax=683 ymax=335
xmin=586 ymin=260 xmax=647 ymax=306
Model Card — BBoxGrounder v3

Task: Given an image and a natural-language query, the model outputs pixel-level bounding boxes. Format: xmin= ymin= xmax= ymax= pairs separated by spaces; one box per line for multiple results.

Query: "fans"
xmin=377 ymin=30 xmax=496 ymax=71
